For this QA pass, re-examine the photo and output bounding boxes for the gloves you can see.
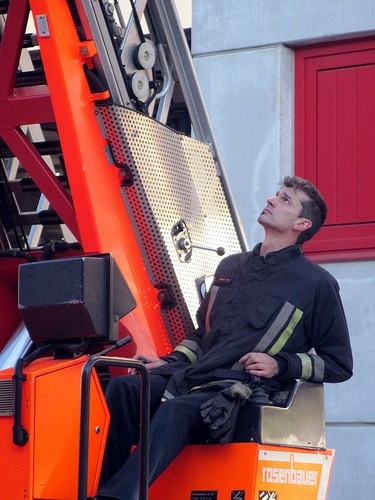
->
[199,383,252,445]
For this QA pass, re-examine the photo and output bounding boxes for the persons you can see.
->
[96,176,353,500]
